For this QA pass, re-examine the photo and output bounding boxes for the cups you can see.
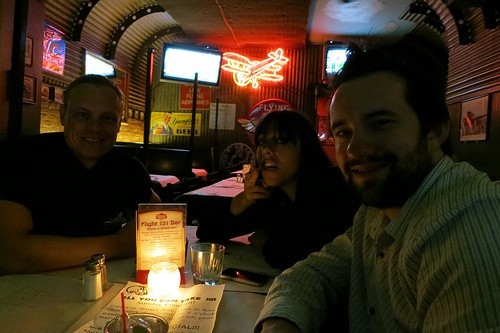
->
[104,313,169,333]
[148,262,181,296]
[242,164,251,182]
[189,244,225,285]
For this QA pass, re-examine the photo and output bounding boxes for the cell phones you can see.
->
[219,267,269,287]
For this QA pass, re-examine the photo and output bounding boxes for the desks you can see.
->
[181,175,248,223]
[0,225,282,333]
[149,173,181,204]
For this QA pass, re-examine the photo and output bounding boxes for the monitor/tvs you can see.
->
[321,41,350,84]
[83,51,114,80]
[159,44,222,88]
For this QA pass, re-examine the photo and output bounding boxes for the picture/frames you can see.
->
[25,35,34,67]
[22,74,38,105]
[459,93,490,142]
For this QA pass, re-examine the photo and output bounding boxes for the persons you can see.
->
[253,48,500,333]
[194,110,362,271]
[0,75,163,275]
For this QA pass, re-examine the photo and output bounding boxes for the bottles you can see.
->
[81,259,104,301]
[237,174,241,183]
[90,254,107,288]
